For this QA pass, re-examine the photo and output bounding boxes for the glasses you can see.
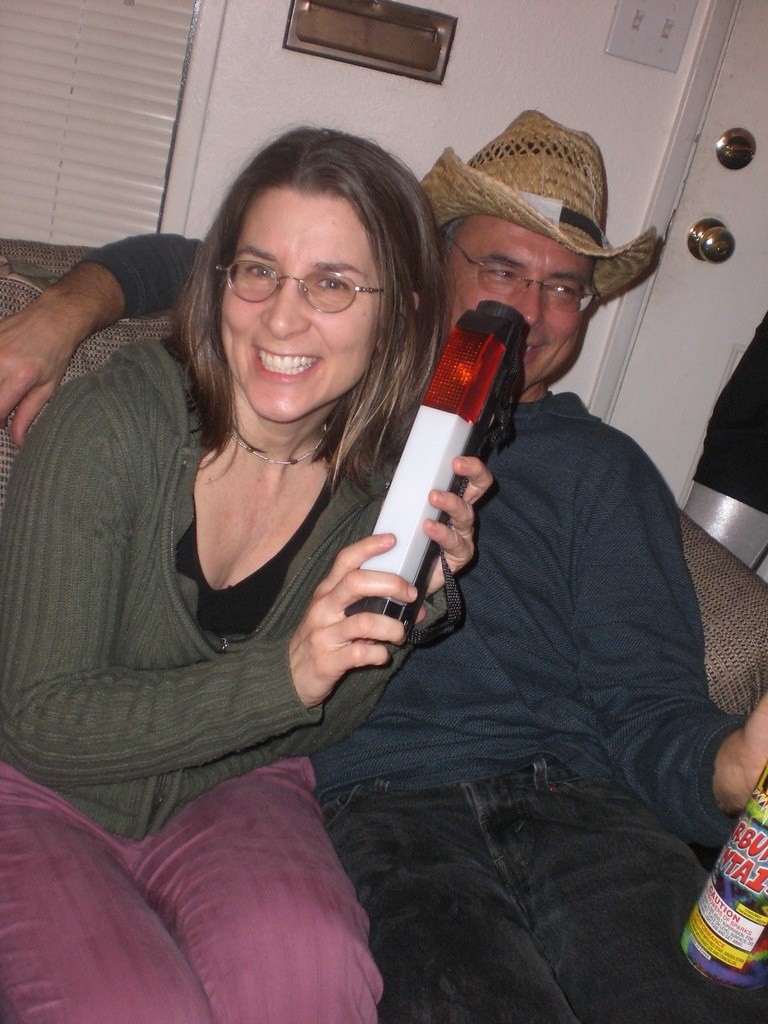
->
[446,234,601,312]
[215,260,383,314]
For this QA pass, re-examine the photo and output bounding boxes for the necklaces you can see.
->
[230,420,328,465]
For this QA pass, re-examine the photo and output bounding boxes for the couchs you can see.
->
[0,239,768,716]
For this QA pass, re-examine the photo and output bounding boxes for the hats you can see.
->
[420,110,657,300]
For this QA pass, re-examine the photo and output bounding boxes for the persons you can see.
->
[0,109,767,1024]
[0,126,494,1024]
